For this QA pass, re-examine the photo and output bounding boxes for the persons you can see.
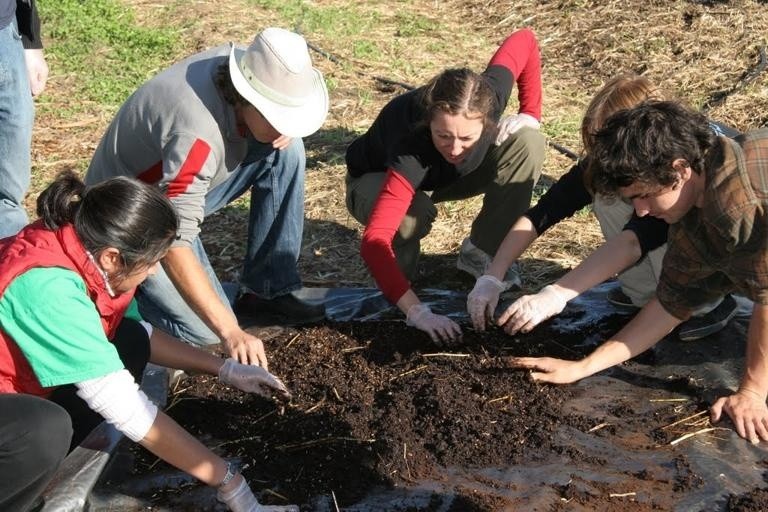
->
[347,24,547,346]
[0,1,50,241]
[463,72,741,345]
[511,88,768,447]
[2,167,300,511]
[83,27,330,370]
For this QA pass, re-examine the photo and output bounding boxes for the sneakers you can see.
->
[372,239,422,289]
[455,235,523,293]
[605,285,642,307]
[676,293,739,343]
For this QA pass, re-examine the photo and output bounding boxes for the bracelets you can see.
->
[213,459,238,491]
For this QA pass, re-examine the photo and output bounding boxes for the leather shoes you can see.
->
[231,288,328,326]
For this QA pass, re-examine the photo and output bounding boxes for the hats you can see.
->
[228,28,330,137]
